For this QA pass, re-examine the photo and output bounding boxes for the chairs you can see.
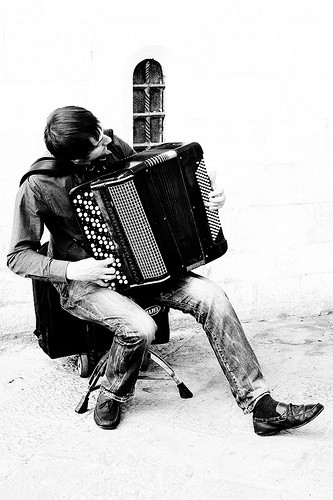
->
[33,242,194,414]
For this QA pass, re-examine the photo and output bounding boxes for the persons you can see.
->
[6,106,325,436]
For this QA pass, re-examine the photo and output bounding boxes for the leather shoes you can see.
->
[94,392,120,430]
[252,403,325,437]
